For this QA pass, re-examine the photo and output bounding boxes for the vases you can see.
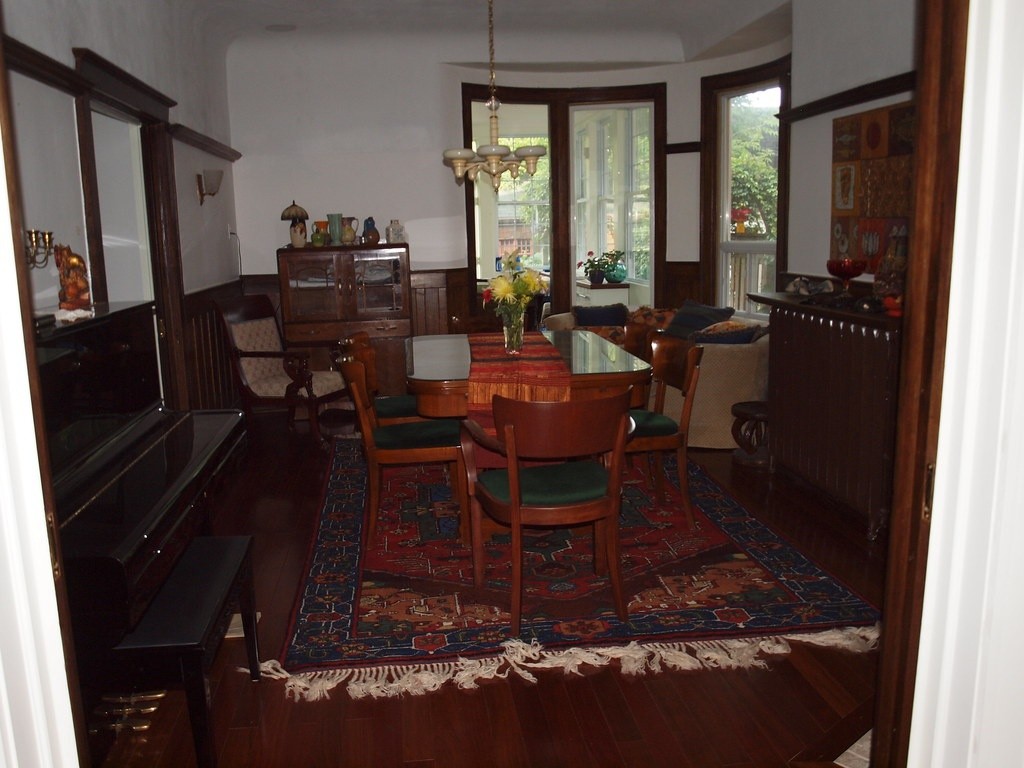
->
[498,303,526,355]
[589,270,605,284]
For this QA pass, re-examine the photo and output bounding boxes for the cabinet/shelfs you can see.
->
[576,280,630,306]
[274,243,411,396]
[748,291,902,541]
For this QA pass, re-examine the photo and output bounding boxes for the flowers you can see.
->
[578,251,606,276]
[483,249,548,348]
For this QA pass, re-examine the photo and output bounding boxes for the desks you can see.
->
[406,331,654,415]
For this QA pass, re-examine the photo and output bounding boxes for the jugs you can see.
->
[312,220,331,246]
[341,217,359,245]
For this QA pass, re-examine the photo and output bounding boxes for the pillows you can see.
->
[570,301,769,344]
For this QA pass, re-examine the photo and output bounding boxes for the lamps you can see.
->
[196,170,224,206]
[26,229,54,267]
[443,0,546,194]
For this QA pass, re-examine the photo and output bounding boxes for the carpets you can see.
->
[239,437,883,702]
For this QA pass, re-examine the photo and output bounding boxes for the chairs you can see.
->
[338,330,457,492]
[604,334,704,525]
[624,321,668,484]
[214,294,360,451]
[335,347,470,550]
[461,385,636,637]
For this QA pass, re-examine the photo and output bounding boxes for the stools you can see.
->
[731,401,771,461]
[114,533,262,768]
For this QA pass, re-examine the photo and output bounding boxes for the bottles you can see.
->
[326,213,344,247]
[386,220,406,244]
[364,217,380,245]
[311,228,323,248]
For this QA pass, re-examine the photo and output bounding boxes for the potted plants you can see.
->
[602,250,626,283]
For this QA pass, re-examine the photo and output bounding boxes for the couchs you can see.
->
[543,301,771,448]
